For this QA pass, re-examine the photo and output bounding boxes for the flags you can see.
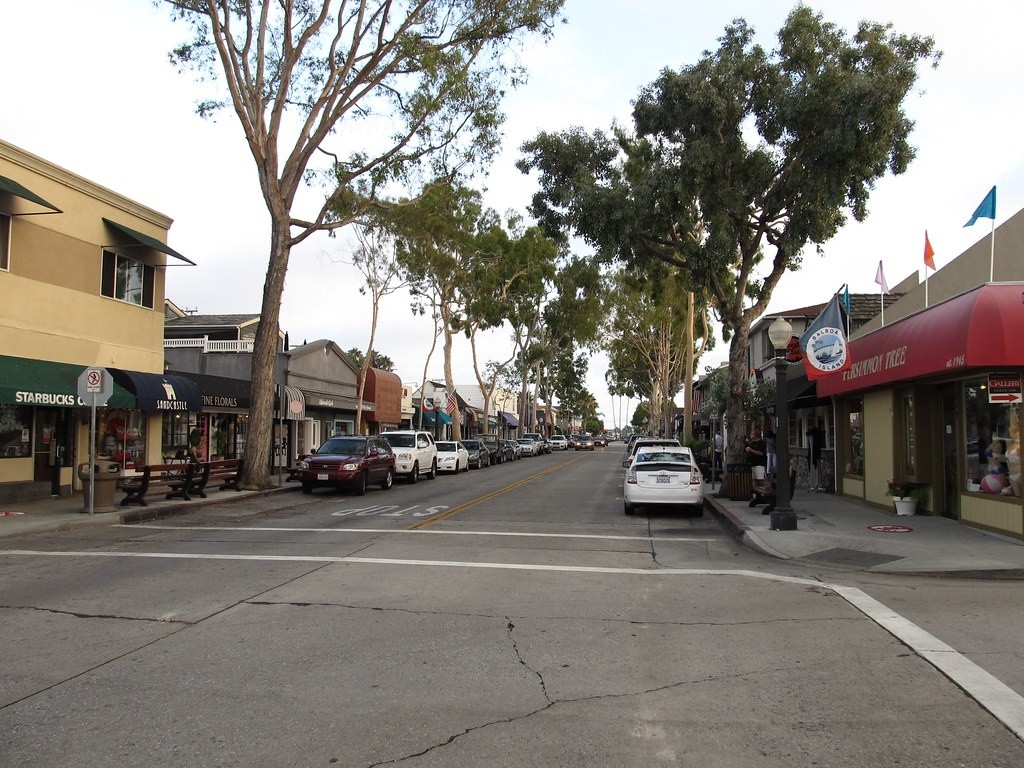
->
[962,186,996,227]
[799,296,853,383]
[875,261,890,296]
[841,285,851,316]
[924,230,937,272]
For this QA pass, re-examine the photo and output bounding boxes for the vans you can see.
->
[565,433,578,447]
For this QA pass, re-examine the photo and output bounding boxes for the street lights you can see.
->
[767,314,798,531]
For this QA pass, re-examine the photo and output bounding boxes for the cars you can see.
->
[461,440,490,470]
[508,439,522,460]
[500,439,514,462]
[543,438,553,454]
[576,435,595,451]
[622,445,706,519]
[434,441,469,475]
[516,438,538,457]
[626,435,653,453]
[550,435,568,450]
[626,438,680,472]
[593,436,608,447]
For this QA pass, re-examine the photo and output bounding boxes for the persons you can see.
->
[711,432,725,470]
[763,429,777,475]
[745,429,767,500]
[983,438,1012,495]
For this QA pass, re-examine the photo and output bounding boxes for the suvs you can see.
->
[474,434,500,464]
[299,433,395,496]
[524,433,544,455]
[380,429,437,483]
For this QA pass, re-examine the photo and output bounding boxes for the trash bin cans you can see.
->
[726,463,754,501]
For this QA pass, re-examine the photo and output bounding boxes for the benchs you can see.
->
[114,462,197,504]
[748,469,796,516]
[191,459,245,498]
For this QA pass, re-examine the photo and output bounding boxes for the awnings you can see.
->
[746,372,832,418]
[300,391,376,412]
[1,354,135,412]
[478,418,496,428]
[814,282,1024,399]
[498,411,520,428]
[436,409,453,425]
[102,367,203,416]
[270,383,305,421]
[411,405,442,428]
[165,370,281,410]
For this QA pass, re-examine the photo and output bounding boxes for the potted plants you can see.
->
[209,432,231,471]
[886,479,926,517]
[191,428,204,462]
